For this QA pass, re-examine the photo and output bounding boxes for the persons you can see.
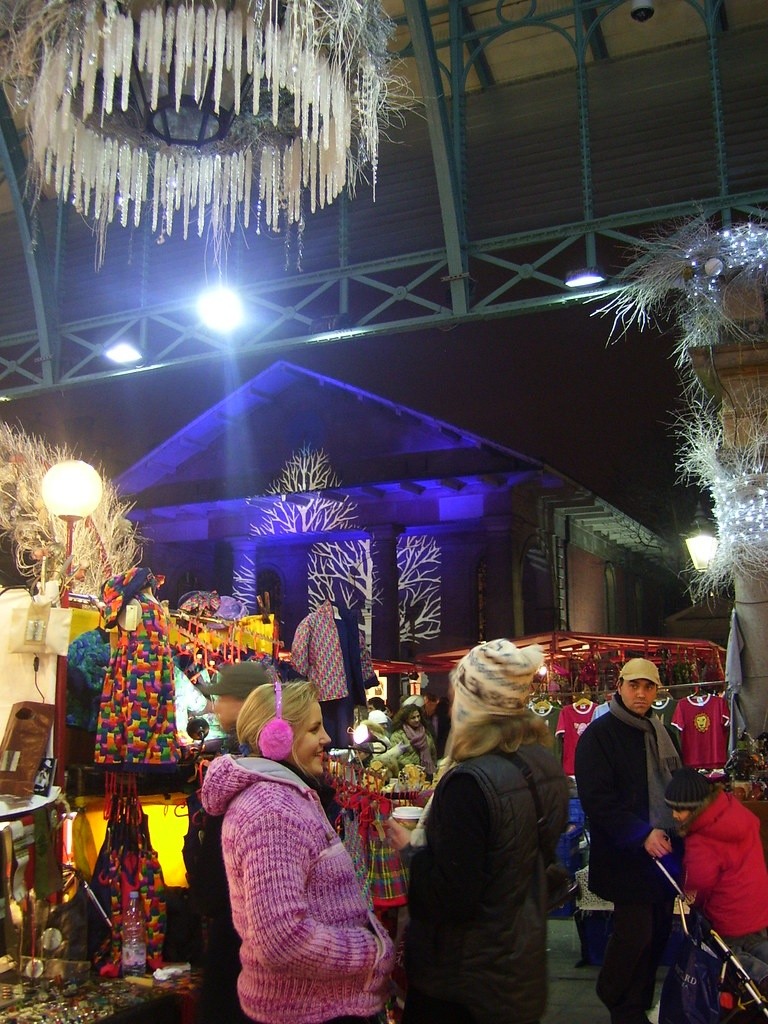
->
[395,637,570,1023]
[345,677,457,791]
[663,767,768,990]
[200,679,397,1024]
[572,657,686,1023]
[183,661,342,1024]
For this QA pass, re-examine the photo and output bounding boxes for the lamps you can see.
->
[0,0,446,276]
[41,459,104,589]
[681,501,720,573]
[102,335,146,369]
[564,265,606,288]
[305,313,354,335]
[187,716,210,763]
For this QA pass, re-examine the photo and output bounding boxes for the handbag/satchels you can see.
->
[0,700,56,796]
[658,933,724,1024]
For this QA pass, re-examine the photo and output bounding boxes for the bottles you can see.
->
[121,891,146,978]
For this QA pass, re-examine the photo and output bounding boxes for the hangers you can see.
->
[655,690,667,700]
[173,618,283,685]
[321,751,425,821]
[102,771,143,827]
[533,696,550,710]
[690,683,708,699]
[574,693,591,707]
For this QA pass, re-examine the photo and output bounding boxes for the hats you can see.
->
[616,657,661,688]
[400,695,425,708]
[201,661,268,697]
[450,639,544,712]
[664,768,710,810]
[368,710,388,723]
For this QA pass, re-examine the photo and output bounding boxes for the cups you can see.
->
[392,807,424,832]
